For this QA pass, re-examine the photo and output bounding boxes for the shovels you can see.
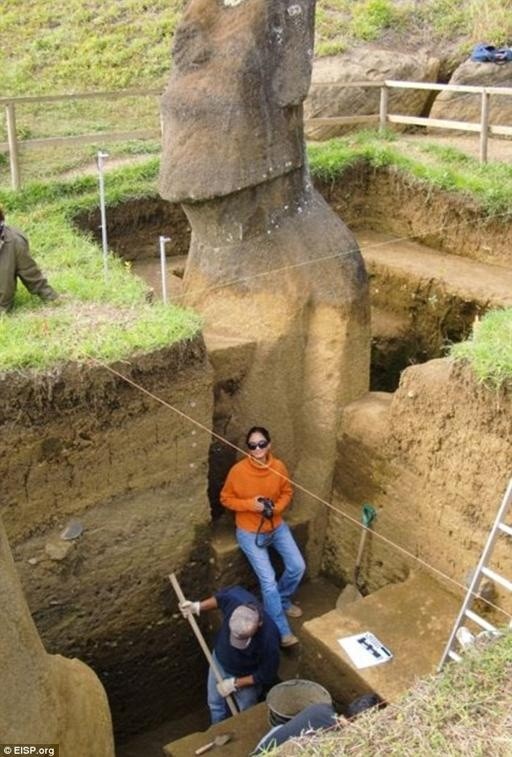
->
[336,505,375,610]
[195,733,231,755]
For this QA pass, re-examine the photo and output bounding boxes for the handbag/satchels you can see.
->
[471,45,512,65]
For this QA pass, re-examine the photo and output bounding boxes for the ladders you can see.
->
[437,477,512,672]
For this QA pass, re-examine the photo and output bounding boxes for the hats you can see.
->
[228,605,258,650]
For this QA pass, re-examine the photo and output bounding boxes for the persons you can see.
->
[219,425,308,649]
[0,206,60,315]
[249,694,385,756]
[179,581,283,724]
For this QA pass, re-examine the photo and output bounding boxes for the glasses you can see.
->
[248,441,268,449]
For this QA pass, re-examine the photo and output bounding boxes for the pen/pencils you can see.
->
[381,647,390,656]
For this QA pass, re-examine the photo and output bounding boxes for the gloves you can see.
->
[216,678,237,696]
[178,601,200,619]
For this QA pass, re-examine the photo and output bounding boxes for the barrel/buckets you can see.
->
[266,677,334,727]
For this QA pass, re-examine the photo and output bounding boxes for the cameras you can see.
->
[257,497,275,519]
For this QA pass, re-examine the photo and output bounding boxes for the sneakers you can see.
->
[285,605,302,617]
[280,635,299,647]
